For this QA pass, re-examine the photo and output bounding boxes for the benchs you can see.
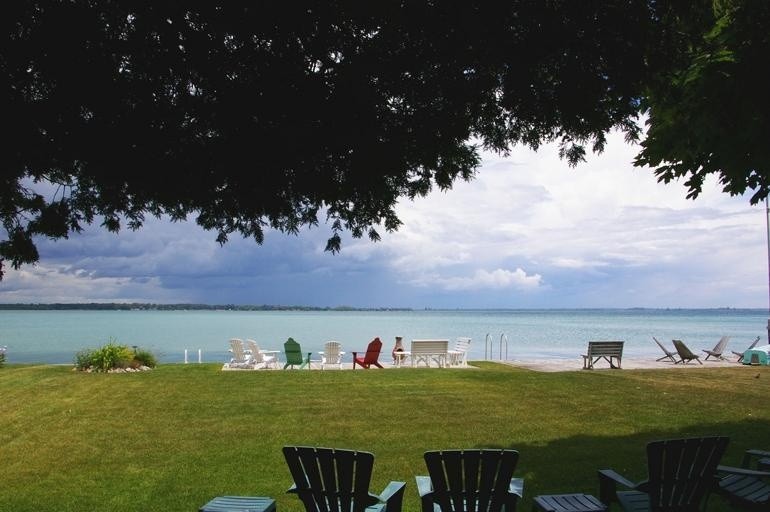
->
[410,340,449,367]
[580,340,625,370]
[447,337,472,367]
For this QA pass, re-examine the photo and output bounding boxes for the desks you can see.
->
[532,493,608,512]
[393,351,411,367]
[199,494,277,512]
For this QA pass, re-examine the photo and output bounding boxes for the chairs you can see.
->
[414,448,524,512]
[711,449,770,512]
[651,334,761,365]
[229,336,385,370]
[597,435,729,512]
[282,446,407,512]
